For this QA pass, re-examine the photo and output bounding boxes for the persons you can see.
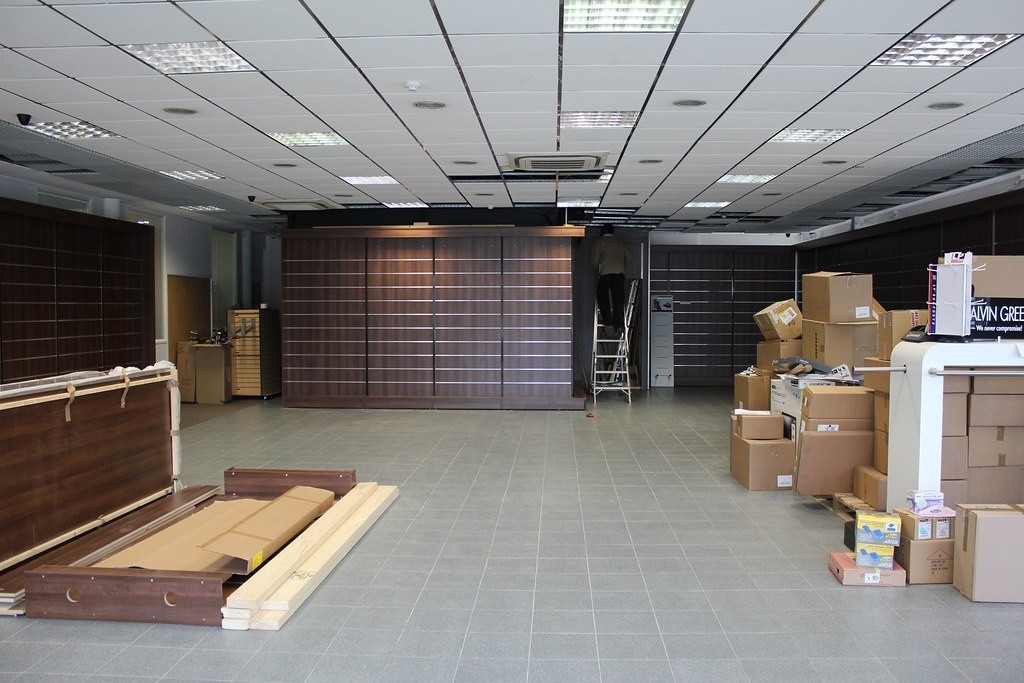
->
[591,223,632,339]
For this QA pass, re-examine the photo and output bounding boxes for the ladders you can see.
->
[609,279,639,390]
[590,278,631,404]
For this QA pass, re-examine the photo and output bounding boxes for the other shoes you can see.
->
[601,320,613,329]
[613,327,623,339]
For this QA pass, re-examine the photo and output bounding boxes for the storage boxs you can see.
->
[177,340,197,404]
[729,272,1024,604]
